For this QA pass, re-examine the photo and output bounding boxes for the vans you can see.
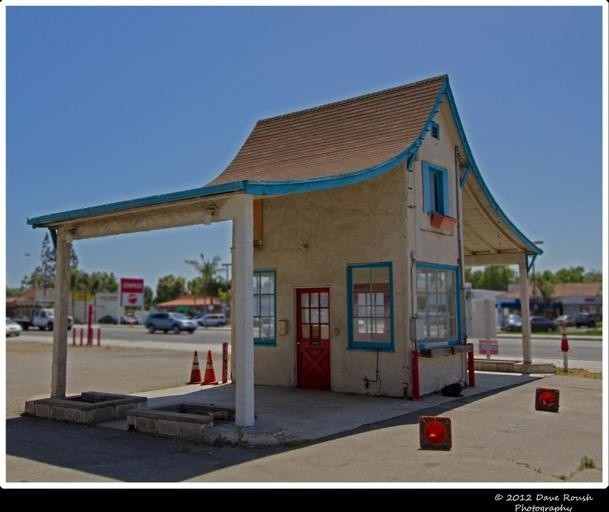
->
[196,313,226,326]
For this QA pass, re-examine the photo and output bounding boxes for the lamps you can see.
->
[203,204,219,226]
[64,226,77,244]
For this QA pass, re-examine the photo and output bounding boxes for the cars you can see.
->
[144,311,198,334]
[98,314,139,324]
[32,308,73,331]
[6,317,23,337]
[500,312,595,332]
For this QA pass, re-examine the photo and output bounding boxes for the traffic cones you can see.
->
[187,350,219,385]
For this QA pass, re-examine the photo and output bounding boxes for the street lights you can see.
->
[221,263,232,303]
